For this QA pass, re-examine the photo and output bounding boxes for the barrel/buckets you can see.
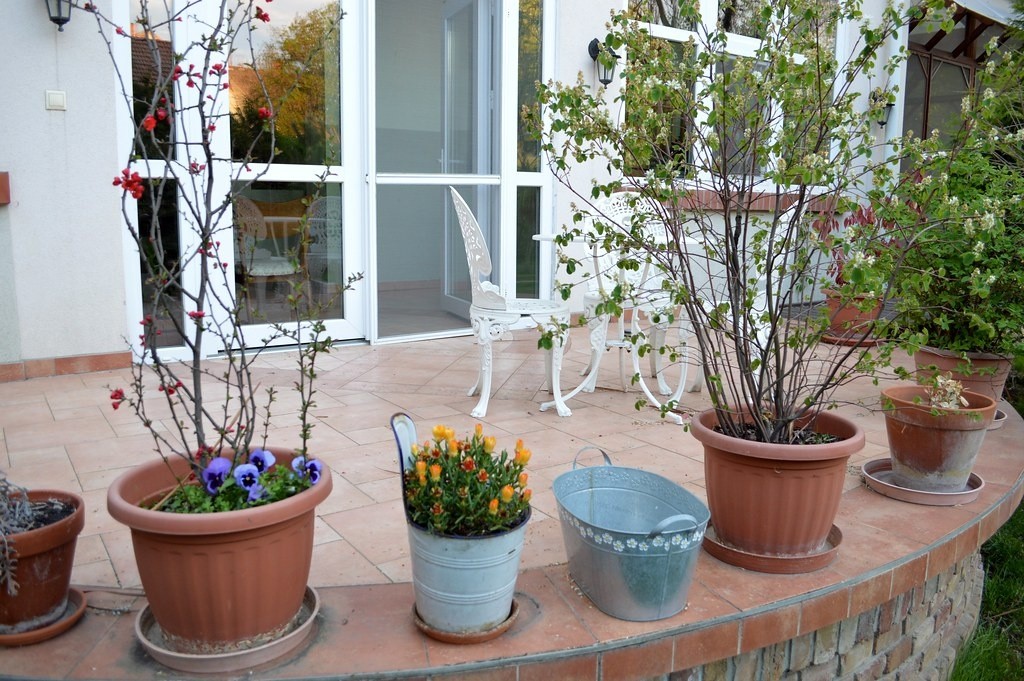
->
[553,447,710,622]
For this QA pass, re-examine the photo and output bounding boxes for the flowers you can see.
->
[63,0,367,513]
[402,423,532,536]
[520,0,1024,444]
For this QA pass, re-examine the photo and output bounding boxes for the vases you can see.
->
[406,492,532,646]
[106,445,334,681]
[688,403,867,575]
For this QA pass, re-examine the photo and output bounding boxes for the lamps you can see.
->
[589,37,622,90]
[45,0,72,32]
[868,91,896,129]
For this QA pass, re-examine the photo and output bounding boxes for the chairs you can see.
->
[449,186,572,419]
[582,190,678,396]
[236,196,319,325]
[664,195,810,410]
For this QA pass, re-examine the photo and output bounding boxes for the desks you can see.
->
[531,232,717,426]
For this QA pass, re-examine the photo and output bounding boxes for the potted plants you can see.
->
[812,170,927,348]
[861,371,996,507]
[886,67,1024,431]
[0,468,88,649]
